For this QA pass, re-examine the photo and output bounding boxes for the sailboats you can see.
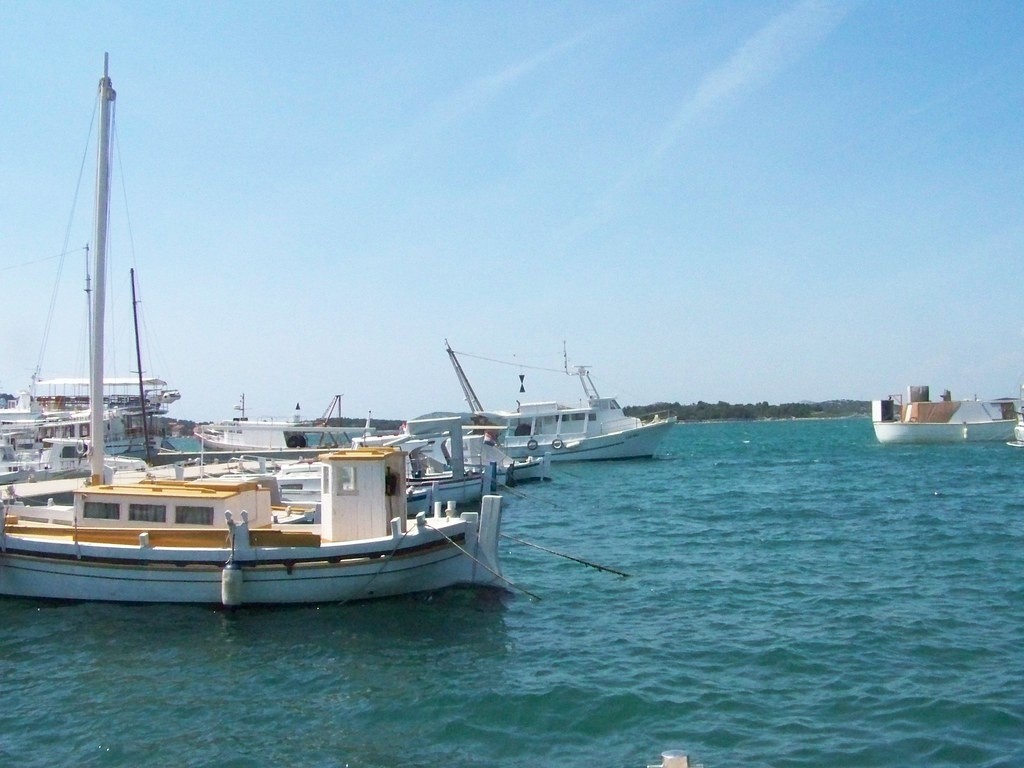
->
[0,49,521,612]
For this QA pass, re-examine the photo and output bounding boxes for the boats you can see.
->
[192,332,679,519]
[871,385,1017,443]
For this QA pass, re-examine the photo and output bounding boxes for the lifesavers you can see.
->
[552,438,563,450]
[75,440,85,455]
[527,439,538,450]
